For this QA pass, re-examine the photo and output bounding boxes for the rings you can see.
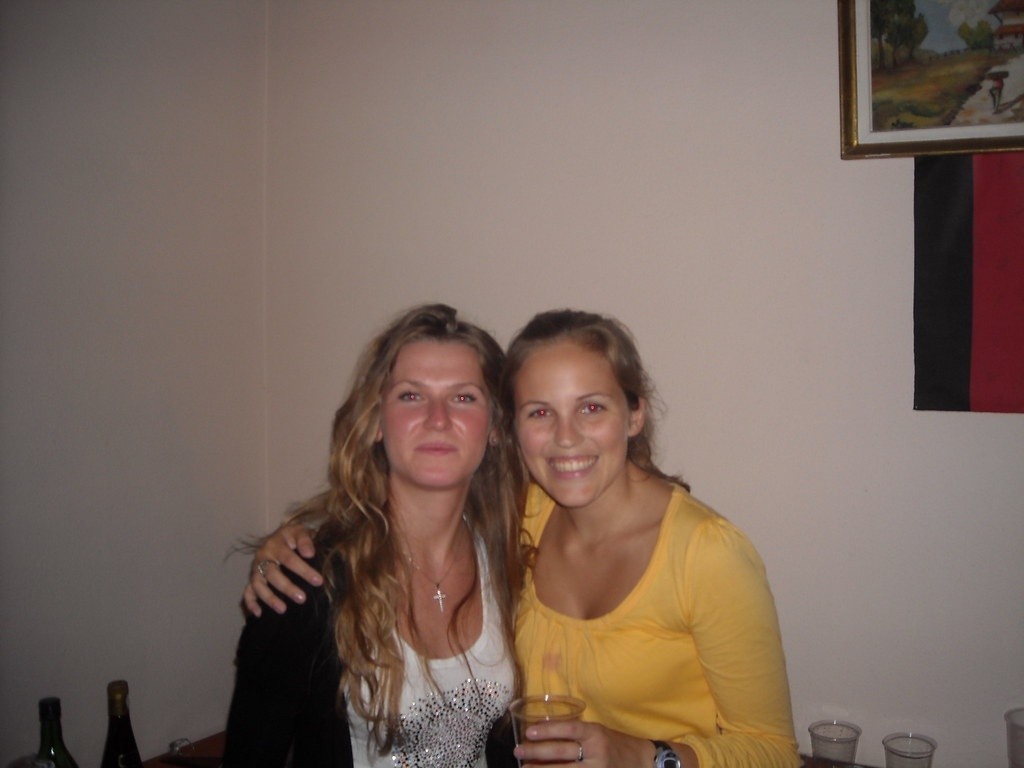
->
[575,741,584,762]
[256,559,280,577]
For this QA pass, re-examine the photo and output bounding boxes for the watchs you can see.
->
[649,739,682,768]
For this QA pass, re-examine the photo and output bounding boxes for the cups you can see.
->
[508,693,587,768]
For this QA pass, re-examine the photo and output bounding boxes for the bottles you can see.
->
[98,678,144,768]
[808,720,861,768]
[32,696,79,768]
[1004,707,1024,768]
[881,731,937,768]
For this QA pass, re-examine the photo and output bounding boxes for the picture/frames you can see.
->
[837,1,1024,161]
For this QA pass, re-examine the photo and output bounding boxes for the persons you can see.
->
[223,303,802,768]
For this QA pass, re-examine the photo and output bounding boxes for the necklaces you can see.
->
[402,520,466,613]
